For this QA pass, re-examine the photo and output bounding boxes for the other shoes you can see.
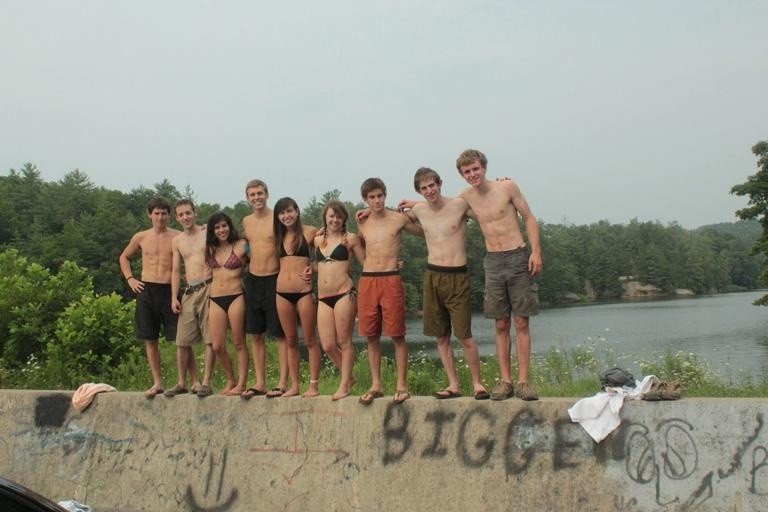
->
[146,385,165,397]
[164,384,188,396]
[197,385,213,396]
[191,384,202,394]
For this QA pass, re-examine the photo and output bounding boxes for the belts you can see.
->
[184,278,213,295]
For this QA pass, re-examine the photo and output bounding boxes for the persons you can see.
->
[239,181,315,399]
[312,201,406,400]
[274,195,319,397]
[204,212,252,396]
[118,196,210,397]
[396,149,543,401]
[354,166,512,399]
[163,200,216,397]
[314,177,425,404]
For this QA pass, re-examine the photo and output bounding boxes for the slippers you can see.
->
[239,387,265,400]
[392,390,411,402]
[359,390,383,403]
[474,390,490,400]
[435,390,462,399]
[266,388,285,398]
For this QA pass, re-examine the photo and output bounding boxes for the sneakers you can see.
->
[643,381,682,401]
[490,384,514,400]
[515,383,538,400]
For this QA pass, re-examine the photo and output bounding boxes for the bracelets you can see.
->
[126,273,134,282]
